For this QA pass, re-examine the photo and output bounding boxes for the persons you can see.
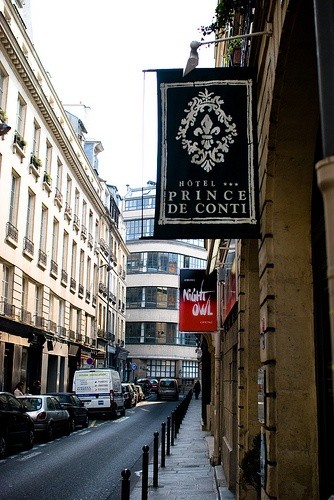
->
[13,382,24,396]
[193,381,201,400]
[27,381,42,395]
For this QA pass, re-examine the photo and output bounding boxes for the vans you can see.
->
[157,378,180,401]
[72,368,126,419]
[137,378,158,392]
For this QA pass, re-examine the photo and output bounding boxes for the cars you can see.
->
[15,395,71,442]
[0,391,35,459]
[43,393,89,431]
[121,382,146,408]
